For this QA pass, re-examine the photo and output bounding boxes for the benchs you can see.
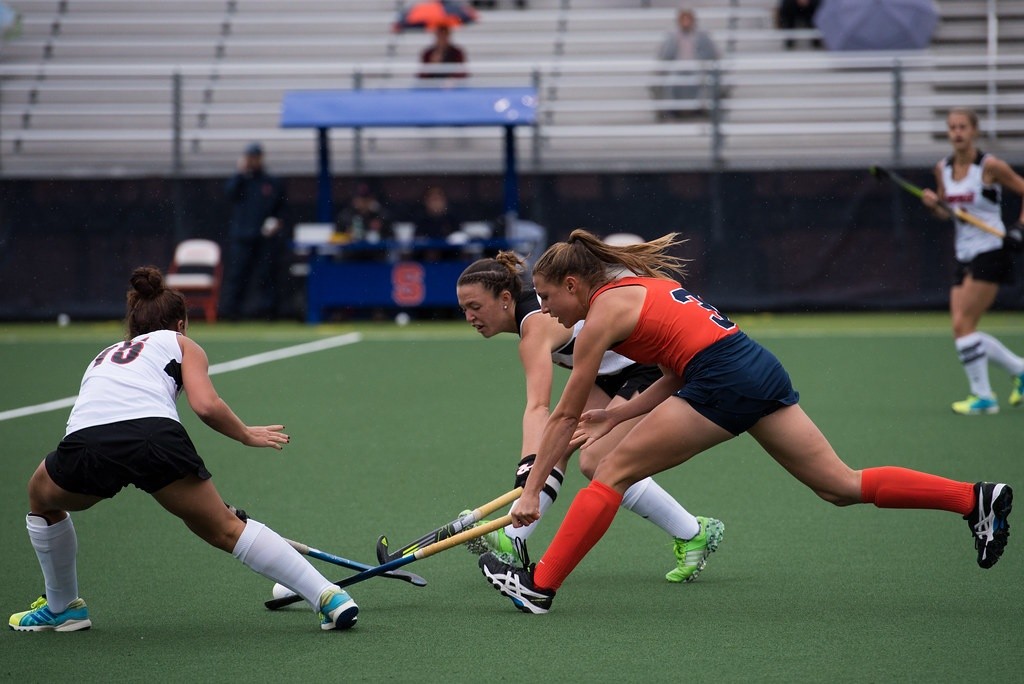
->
[290,223,547,297]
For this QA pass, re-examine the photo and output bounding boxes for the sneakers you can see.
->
[478,536,557,615]
[951,394,1000,415]
[962,481,1013,569]
[458,510,523,567]
[8,594,91,632]
[1009,370,1024,407]
[318,587,359,630]
[665,516,725,583]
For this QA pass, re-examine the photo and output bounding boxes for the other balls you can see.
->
[272,583,297,599]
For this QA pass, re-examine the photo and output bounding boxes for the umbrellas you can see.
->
[813,0,939,51]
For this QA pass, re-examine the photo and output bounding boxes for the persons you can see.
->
[456,252,724,583]
[419,24,466,78]
[479,229,1013,614]
[337,183,395,319]
[774,0,821,50]
[8,265,359,633]
[414,186,456,261]
[220,143,286,317]
[923,110,1024,415]
[648,12,728,118]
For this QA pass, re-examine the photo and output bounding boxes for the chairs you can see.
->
[163,237,223,323]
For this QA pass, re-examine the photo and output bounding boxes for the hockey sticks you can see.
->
[377,484,524,571]
[282,537,428,587]
[264,514,513,610]
[870,160,1006,239]
